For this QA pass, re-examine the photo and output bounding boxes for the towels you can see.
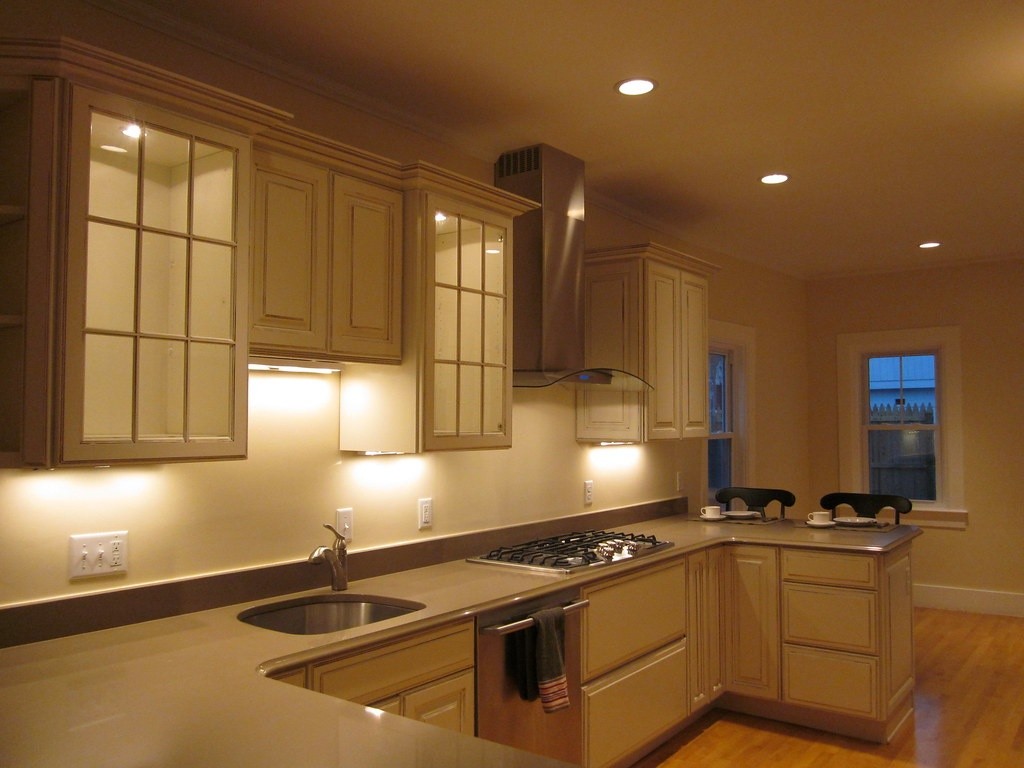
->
[516,604,571,713]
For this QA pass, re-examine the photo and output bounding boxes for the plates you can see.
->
[806,521,835,527]
[721,512,761,518]
[834,516,877,526]
[700,515,726,521]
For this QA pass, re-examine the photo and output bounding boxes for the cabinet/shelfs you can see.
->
[781,542,916,720]
[719,541,778,704]
[580,557,691,768]
[1,35,296,476]
[687,546,724,709]
[251,123,407,378]
[337,158,542,464]
[578,241,722,448]
[307,615,476,740]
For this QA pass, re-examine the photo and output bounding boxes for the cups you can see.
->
[808,511,829,523]
[701,506,720,516]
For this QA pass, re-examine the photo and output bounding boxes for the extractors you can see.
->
[495,144,653,392]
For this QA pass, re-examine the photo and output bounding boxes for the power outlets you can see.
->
[70,530,129,584]
[418,498,434,531]
[583,480,593,506]
[677,471,683,491]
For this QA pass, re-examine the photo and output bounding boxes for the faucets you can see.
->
[308,523,352,591]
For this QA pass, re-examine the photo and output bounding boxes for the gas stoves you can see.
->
[464,524,674,576]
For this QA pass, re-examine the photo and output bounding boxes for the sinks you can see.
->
[236,591,427,636]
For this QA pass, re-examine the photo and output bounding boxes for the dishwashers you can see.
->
[476,587,589,768]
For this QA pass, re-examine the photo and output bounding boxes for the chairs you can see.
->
[715,486,796,519]
[821,490,912,525]
